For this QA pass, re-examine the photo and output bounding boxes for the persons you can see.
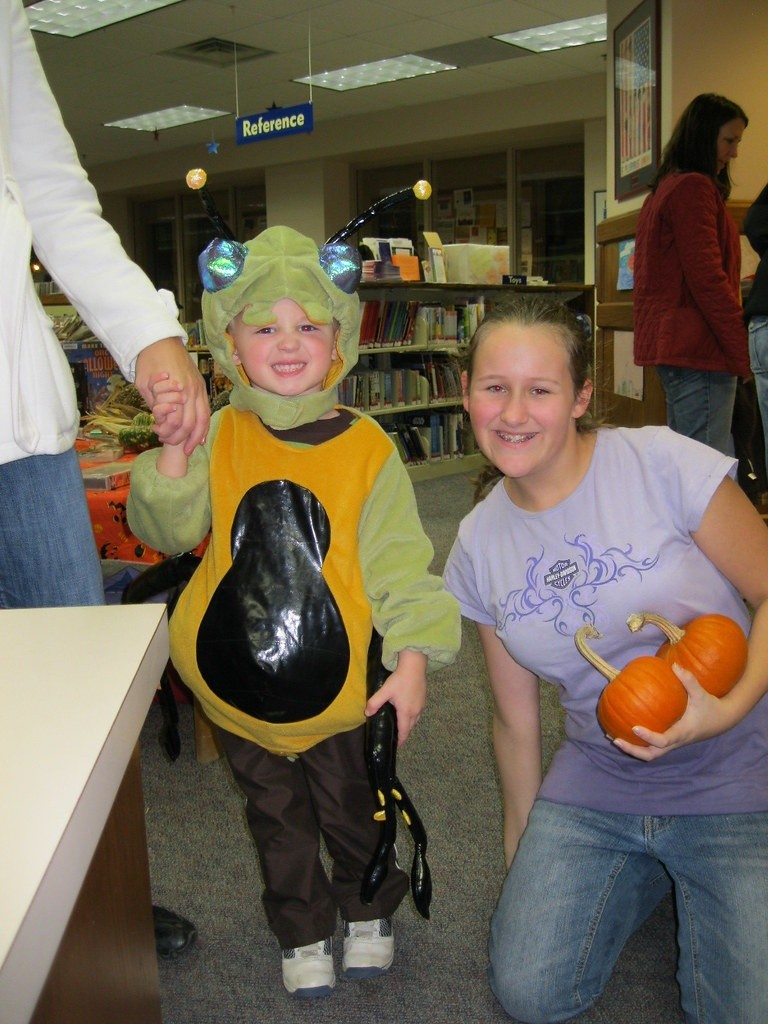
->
[126,225,462,998]
[633,92,768,482]
[0,0,211,956]
[443,296,768,1024]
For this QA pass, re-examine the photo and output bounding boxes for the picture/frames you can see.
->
[612,0,662,199]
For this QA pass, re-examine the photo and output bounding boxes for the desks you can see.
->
[0,591,171,1024]
[72,439,210,563]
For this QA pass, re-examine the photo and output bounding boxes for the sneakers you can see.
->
[282,935,334,995]
[341,919,395,977]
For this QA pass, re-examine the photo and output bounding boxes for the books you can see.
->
[49,312,100,341]
[34,281,64,297]
[182,318,234,405]
[338,238,549,469]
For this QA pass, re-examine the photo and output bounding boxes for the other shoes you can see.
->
[153,905,195,958]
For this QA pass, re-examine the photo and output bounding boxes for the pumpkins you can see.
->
[574,626,687,748]
[627,612,747,698]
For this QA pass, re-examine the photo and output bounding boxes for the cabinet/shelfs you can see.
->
[60,280,599,488]
